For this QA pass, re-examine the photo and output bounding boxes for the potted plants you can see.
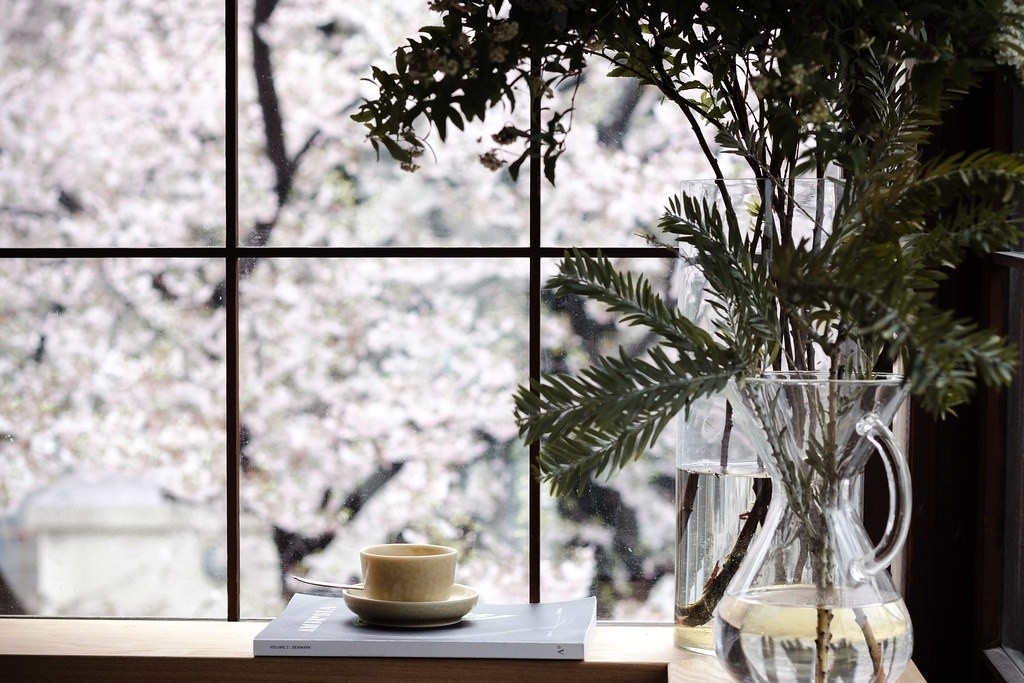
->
[350,0,1024,683]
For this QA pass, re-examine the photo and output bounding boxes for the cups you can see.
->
[359,544,459,602]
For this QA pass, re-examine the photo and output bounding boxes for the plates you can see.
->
[343,581,481,627]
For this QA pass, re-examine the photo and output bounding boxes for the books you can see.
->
[254,592,597,659]
